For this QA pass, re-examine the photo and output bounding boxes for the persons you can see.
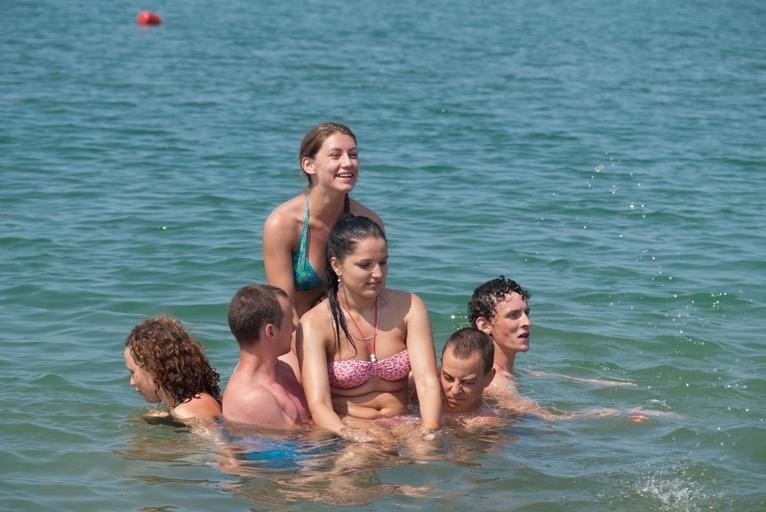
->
[222,284,342,473]
[294,212,445,503]
[123,312,252,474]
[261,120,386,382]
[439,327,505,436]
[467,274,688,422]
